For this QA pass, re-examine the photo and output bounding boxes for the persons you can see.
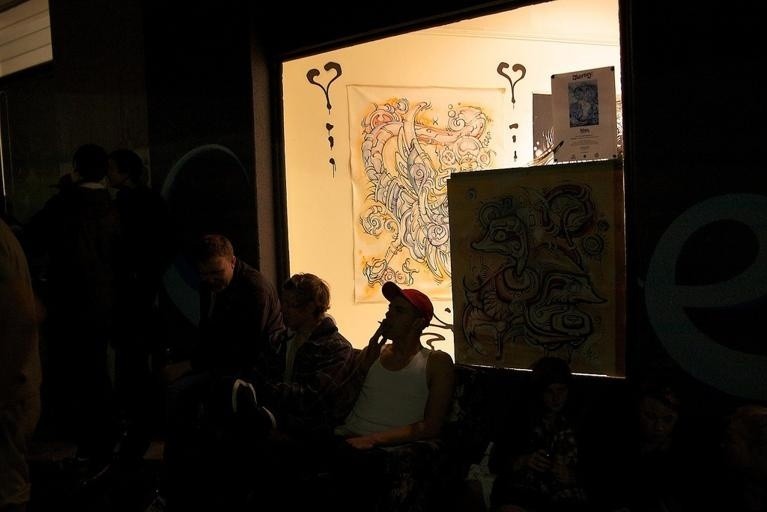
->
[39,143,112,225]
[193,234,285,348]
[727,406,767,481]
[103,147,149,198]
[323,281,454,459]
[0,217,46,512]
[490,356,607,512]
[632,378,683,443]
[217,273,355,436]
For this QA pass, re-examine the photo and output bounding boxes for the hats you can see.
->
[382,282,433,326]
